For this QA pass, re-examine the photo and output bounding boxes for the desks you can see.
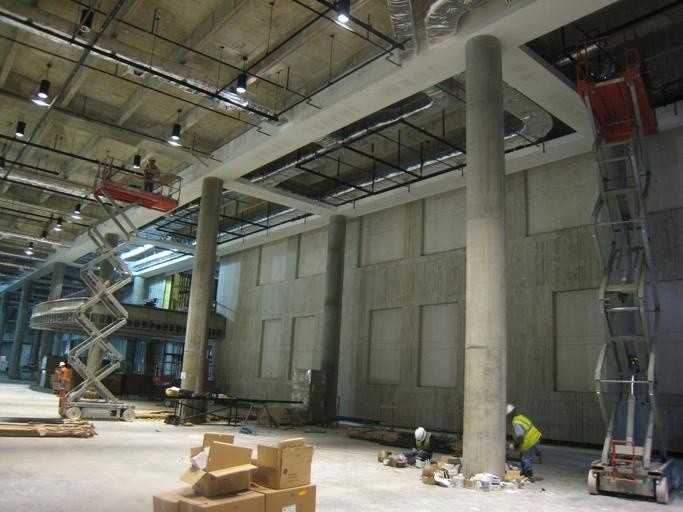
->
[162,395,236,427]
[237,398,303,429]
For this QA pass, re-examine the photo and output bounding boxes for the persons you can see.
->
[399,427,436,465]
[54,362,67,397]
[143,156,160,193]
[506,403,542,483]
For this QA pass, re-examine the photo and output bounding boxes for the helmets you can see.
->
[415,426,426,442]
[507,404,515,416]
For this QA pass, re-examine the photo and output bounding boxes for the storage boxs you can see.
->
[151,431,317,512]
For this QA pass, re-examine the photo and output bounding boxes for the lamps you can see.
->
[57,217,63,227]
[74,203,81,214]
[133,155,141,170]
[336,2,351,23]
[171,123,181,141]
[236,72,247,94]
[15,121,26,137]
[40,230,47,242]
[38,79,51,99]
[78,7,95,33]
[29,241,33,250]
